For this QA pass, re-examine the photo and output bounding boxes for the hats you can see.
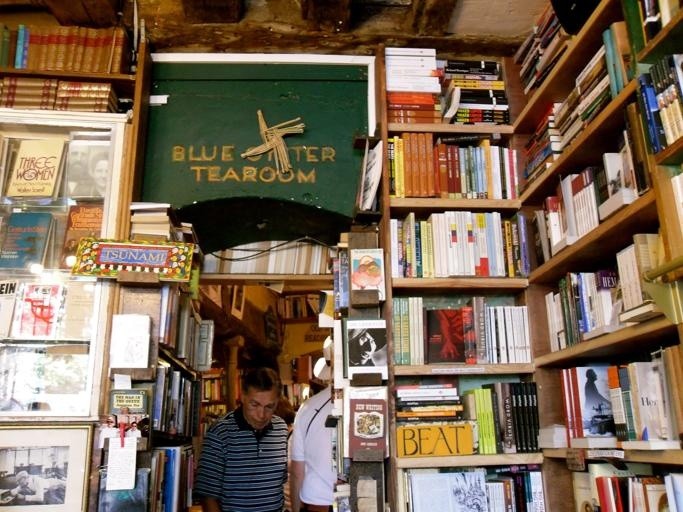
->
[313,336,333,380]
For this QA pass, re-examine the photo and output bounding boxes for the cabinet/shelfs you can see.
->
[197,363,245,434]
[99,192,206,512]
[371,46,548,512]
[0,27,146,512]
[509,0,682,512]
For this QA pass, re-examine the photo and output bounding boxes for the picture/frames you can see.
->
[0,425,91,512]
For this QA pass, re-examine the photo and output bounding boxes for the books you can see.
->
[514,0,682,512]
[2,19,131,203]
[1,201,214,511]
[331,47,546,511]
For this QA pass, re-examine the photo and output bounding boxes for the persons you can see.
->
[192,365,289,512]
[286,329,335,512]
